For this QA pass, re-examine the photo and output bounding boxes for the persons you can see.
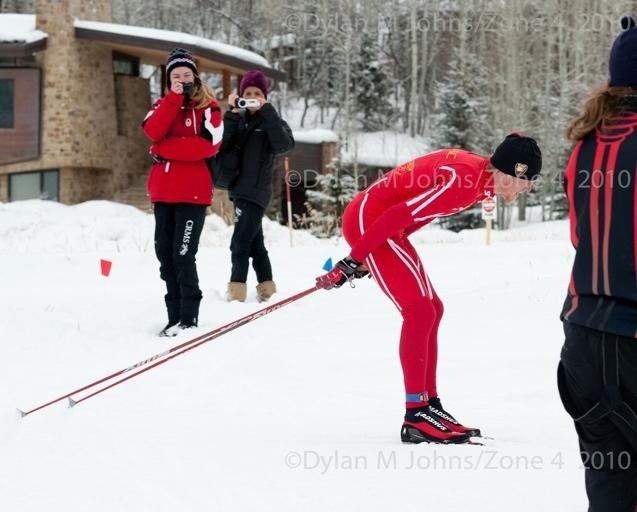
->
[139,45,224,338]
[314,132,543,446]
[221,70,297,305]
[555,15,637,511]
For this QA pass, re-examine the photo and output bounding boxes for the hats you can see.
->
[240,70,267,97]
[165,46,197,75]
[490,132,542,180]
[608,27,637,89]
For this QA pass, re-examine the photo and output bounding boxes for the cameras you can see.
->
[235,97,260,109]
[181,81,194,98]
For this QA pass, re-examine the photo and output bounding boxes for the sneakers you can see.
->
[400,398,480,445]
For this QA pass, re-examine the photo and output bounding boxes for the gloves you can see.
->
[316,257,369,290]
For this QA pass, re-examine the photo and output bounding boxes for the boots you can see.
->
[161,292,202,336]
[256,280,276,303]
[226,282,247,302]
